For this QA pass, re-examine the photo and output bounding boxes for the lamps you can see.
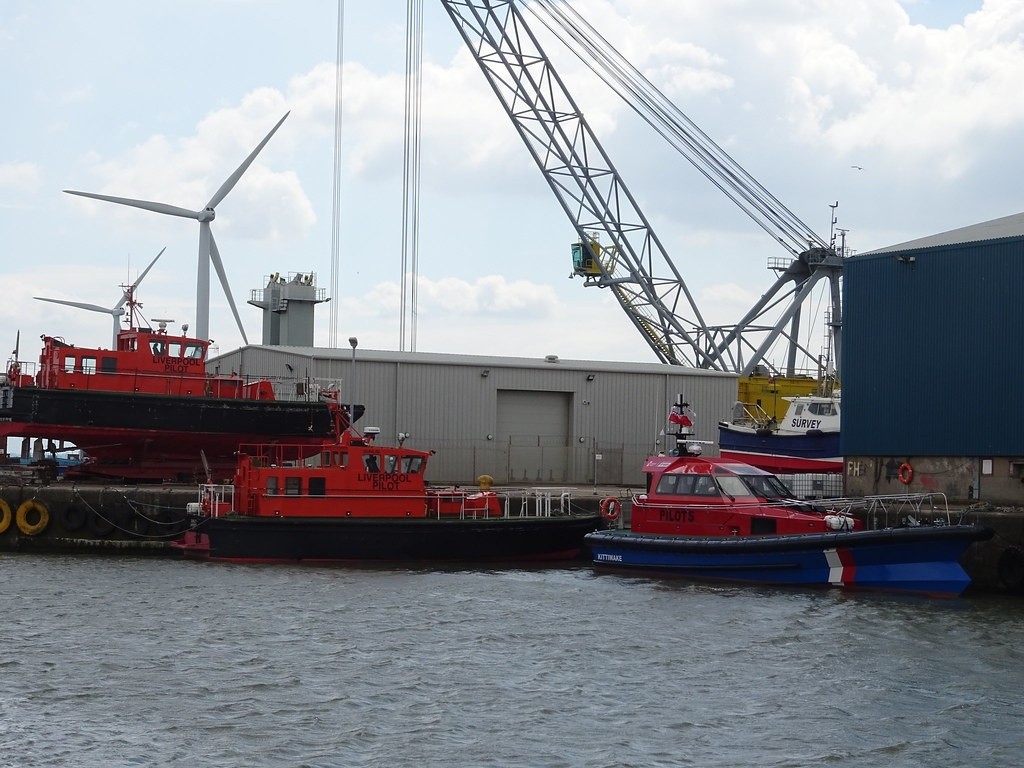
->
[481,369,489,377]
[891,253,910,262]
[586,375,594,381]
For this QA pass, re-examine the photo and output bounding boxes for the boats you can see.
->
[718,352,845,478]
[582,393,996,598]
[164,424,622,565]
[0,249,366,484]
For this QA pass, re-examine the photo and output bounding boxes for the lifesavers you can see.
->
[58,502,87,532]
[88,506,116,538]
[898,463,913,484]
[0,499,11,533]
[478,475,493,491]
[15,498,51,538]
[602,498,621,519]
[120,513,150,539]
[155,510,182,540]
